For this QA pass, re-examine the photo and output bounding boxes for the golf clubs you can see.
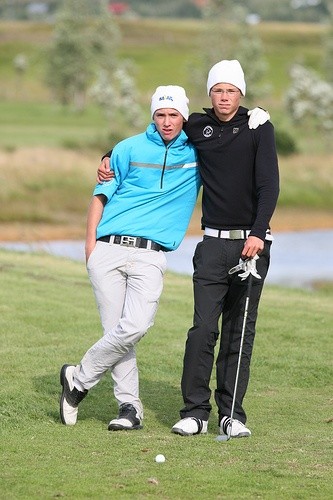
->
[216,260,257,442]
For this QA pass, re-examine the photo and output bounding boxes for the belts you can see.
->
[204,225,274,244]
[97,233,171,253]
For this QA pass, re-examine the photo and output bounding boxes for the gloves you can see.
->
[246,105,270,131]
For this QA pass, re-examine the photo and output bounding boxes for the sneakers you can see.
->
[56,364,89,428]
[106,401,145,433]
[171,414,210,437]
[217,412,251,440]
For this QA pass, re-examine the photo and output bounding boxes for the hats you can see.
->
[150,84,190,121]
[206,58,246,98]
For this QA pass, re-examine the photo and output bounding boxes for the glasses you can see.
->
[212,87,238,96]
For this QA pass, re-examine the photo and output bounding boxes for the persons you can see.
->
[60,85,270,431]
[96,59,280,438]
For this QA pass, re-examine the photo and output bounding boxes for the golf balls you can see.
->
[155,454,165,463]
